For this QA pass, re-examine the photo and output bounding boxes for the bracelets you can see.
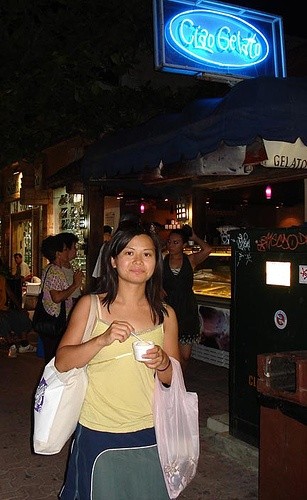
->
[157,359,171,371]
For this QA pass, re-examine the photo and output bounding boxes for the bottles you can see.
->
[74,268,89,291]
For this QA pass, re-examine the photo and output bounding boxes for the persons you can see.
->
[13,253,31,278]
[53,224,180,500]
[39,233,86,363]
[162,225,212,360]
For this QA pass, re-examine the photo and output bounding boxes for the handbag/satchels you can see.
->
[32,300,67,340]
[32,293,98,455]
[154,357,199,498]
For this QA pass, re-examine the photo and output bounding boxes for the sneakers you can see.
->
[8,345,17,358]
[18,344,37,353]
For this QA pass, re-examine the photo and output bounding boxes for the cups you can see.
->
[164,220,181,230]
[132,340,155,363]
